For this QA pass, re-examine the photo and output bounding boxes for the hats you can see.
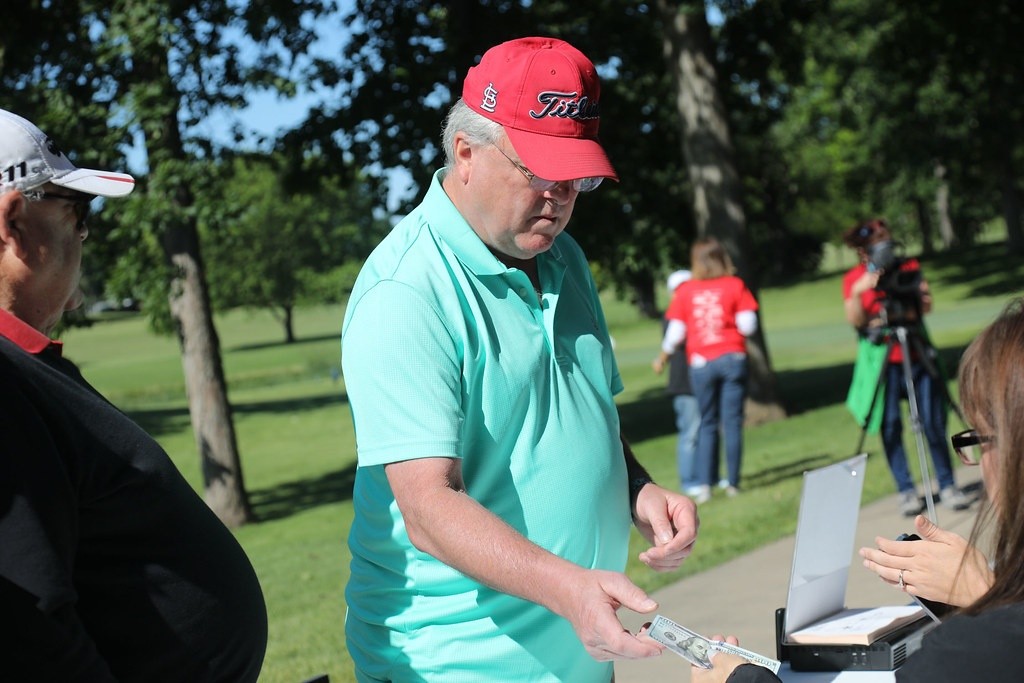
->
[462,37,619,183]
[0,109,135,198]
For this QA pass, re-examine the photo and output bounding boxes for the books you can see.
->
[781,454,928,647]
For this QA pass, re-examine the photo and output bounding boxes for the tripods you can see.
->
[855,295,969,526]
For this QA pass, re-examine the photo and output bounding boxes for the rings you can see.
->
[898,568,907,589]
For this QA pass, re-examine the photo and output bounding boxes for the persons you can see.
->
[342,35,702,681]
[662,266,700,498]
[1,107,271,683]
[647,231,760,502]
[690,298,1024,683]
[843,216,972,519]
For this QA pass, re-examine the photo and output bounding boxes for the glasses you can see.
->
[23,190,91,231]
[488,138,605,193]
[951,429,997,465]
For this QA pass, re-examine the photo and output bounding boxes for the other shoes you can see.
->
[939,484,969,509]
[902,491,924,515]
